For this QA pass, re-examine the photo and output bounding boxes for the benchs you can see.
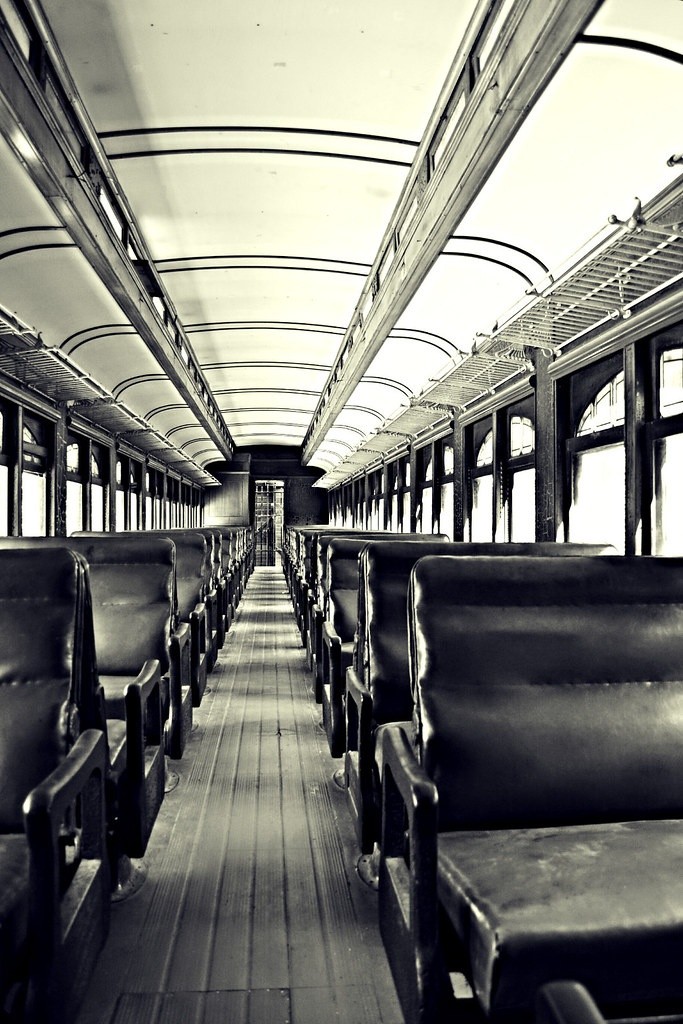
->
[0,523,255,1024]
[279,520,682,1023]
